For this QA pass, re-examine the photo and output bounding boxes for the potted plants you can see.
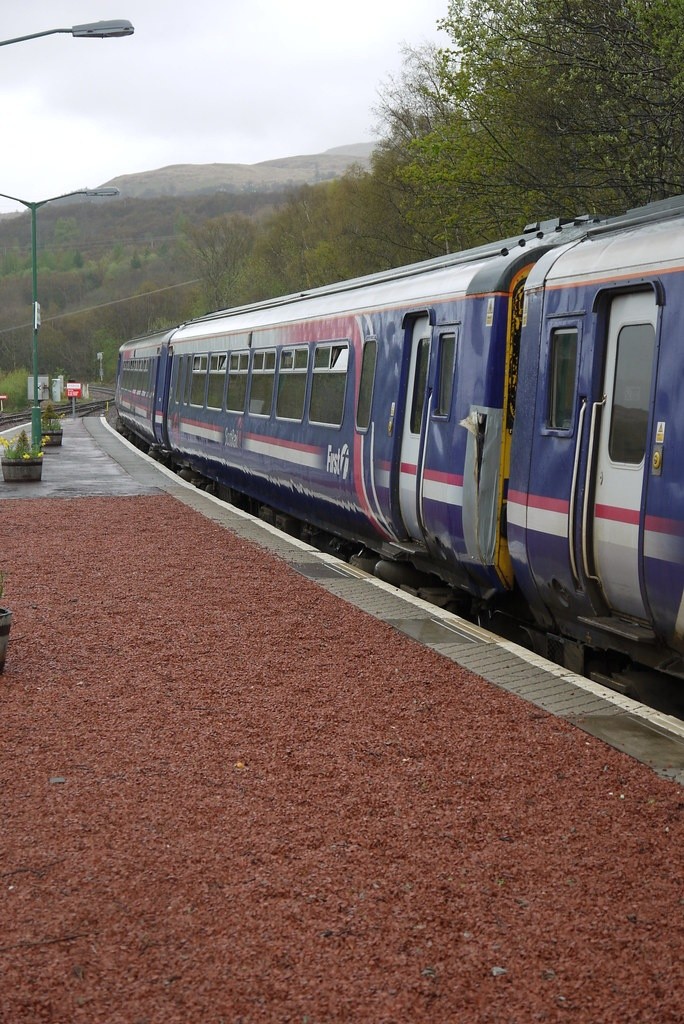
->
[0,567,13,678]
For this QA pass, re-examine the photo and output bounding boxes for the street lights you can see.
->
[0,186,119,473]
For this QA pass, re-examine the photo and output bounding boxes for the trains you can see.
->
[115,192,684,708]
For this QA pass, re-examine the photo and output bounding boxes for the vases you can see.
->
[1,457,45,482]
[41,429,63,446]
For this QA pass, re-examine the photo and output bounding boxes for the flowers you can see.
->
[0,430,50,462]
[41,403,65,430]
